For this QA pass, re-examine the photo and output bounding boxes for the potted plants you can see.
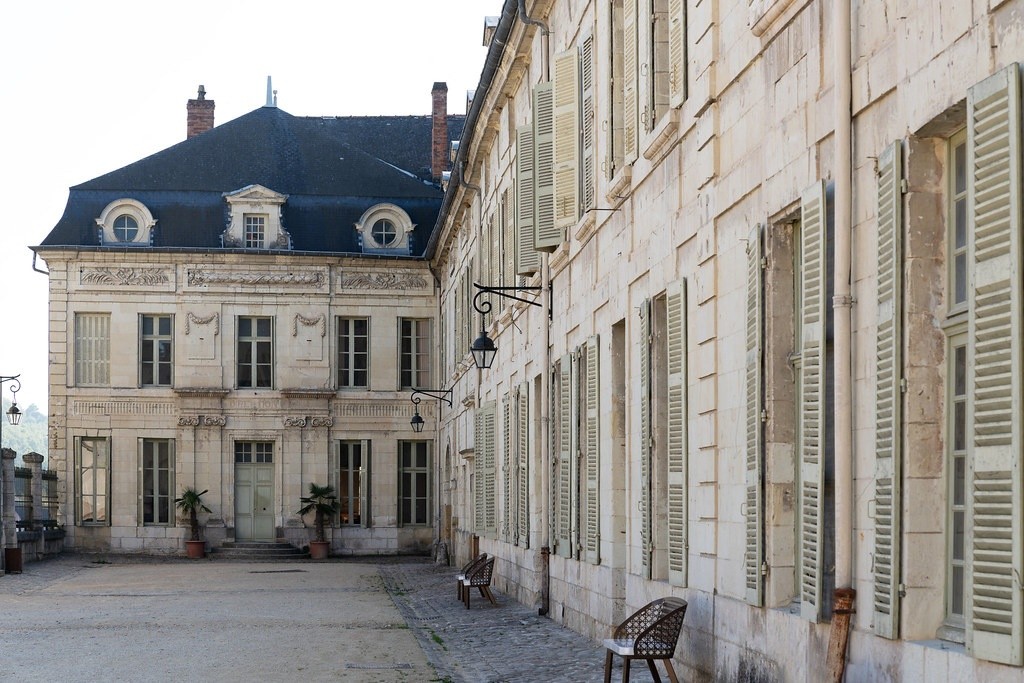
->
[172,485,214,559]
[296,481,342,560]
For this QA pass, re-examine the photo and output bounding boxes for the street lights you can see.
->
[0,374,22,449]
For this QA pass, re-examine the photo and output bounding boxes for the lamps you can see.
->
[409,387,453,432]
[469,278,554,370]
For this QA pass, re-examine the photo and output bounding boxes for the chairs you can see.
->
[600,596,688,683]
[462,556,498,610]
[454,552,488,602]
